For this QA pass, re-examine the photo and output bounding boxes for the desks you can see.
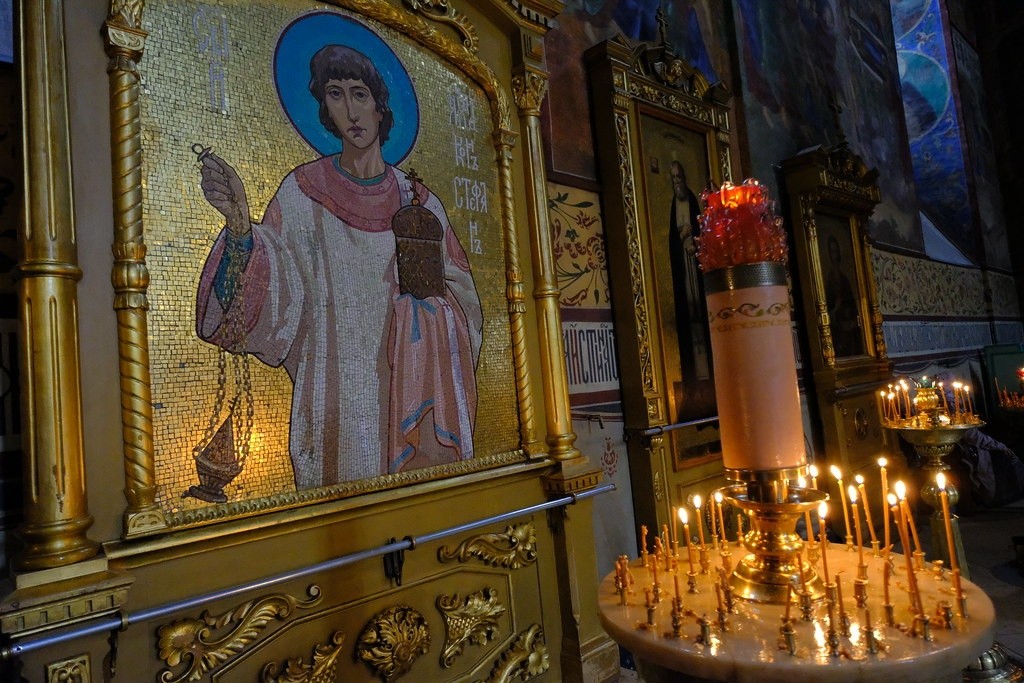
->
[595,538,998,683]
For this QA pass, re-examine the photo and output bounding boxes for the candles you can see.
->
[879,375,973,423]
[614,455,965,634]
